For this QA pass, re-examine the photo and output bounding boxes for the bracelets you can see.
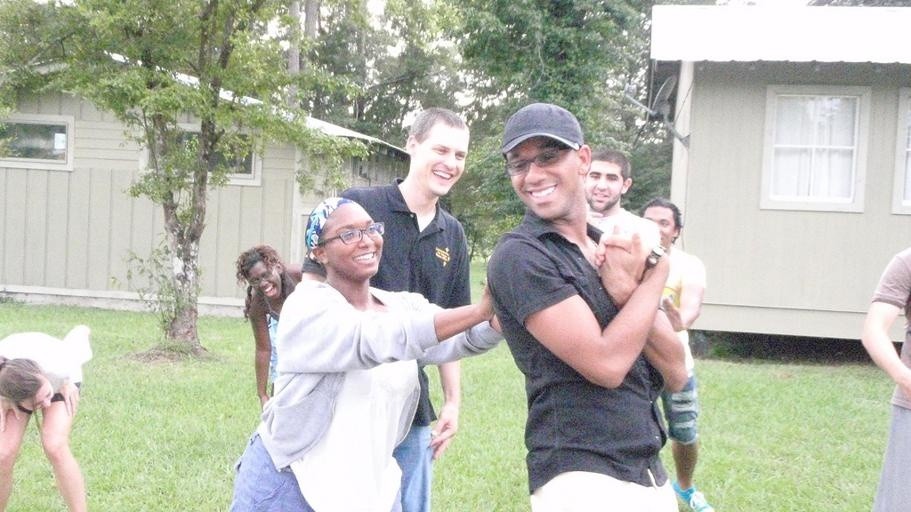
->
[640,247,663,282]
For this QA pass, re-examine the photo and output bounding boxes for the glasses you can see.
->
[504,146,569,176]
[317,221,385,246]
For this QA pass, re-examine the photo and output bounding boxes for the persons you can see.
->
[332,107,471,511]
[585,147,662,259]
[232,195,506,512]
[637,196,715,512]
[0,322,93,511]
[859,246,911,511]
[487,101,692,512]
[234,244,302,412]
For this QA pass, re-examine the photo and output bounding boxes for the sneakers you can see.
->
[672,480,717,511]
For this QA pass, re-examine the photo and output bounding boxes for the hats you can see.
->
[502,102,584,156]
